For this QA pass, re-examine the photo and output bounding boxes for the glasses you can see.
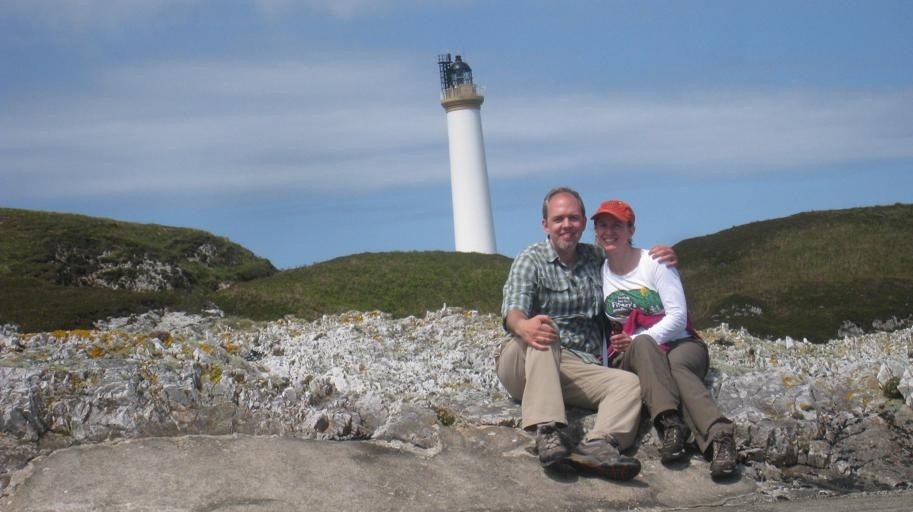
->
[610,321,622,335]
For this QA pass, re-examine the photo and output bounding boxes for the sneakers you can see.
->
[658,418,695,466]
[564,433,642,482]
[533,420,568,468]
[710,431,739,479]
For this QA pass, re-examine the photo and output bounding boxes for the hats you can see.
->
[590,199,636,225]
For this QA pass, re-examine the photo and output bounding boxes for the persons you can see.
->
[495,187,678,482]
[590,200,739,482]
